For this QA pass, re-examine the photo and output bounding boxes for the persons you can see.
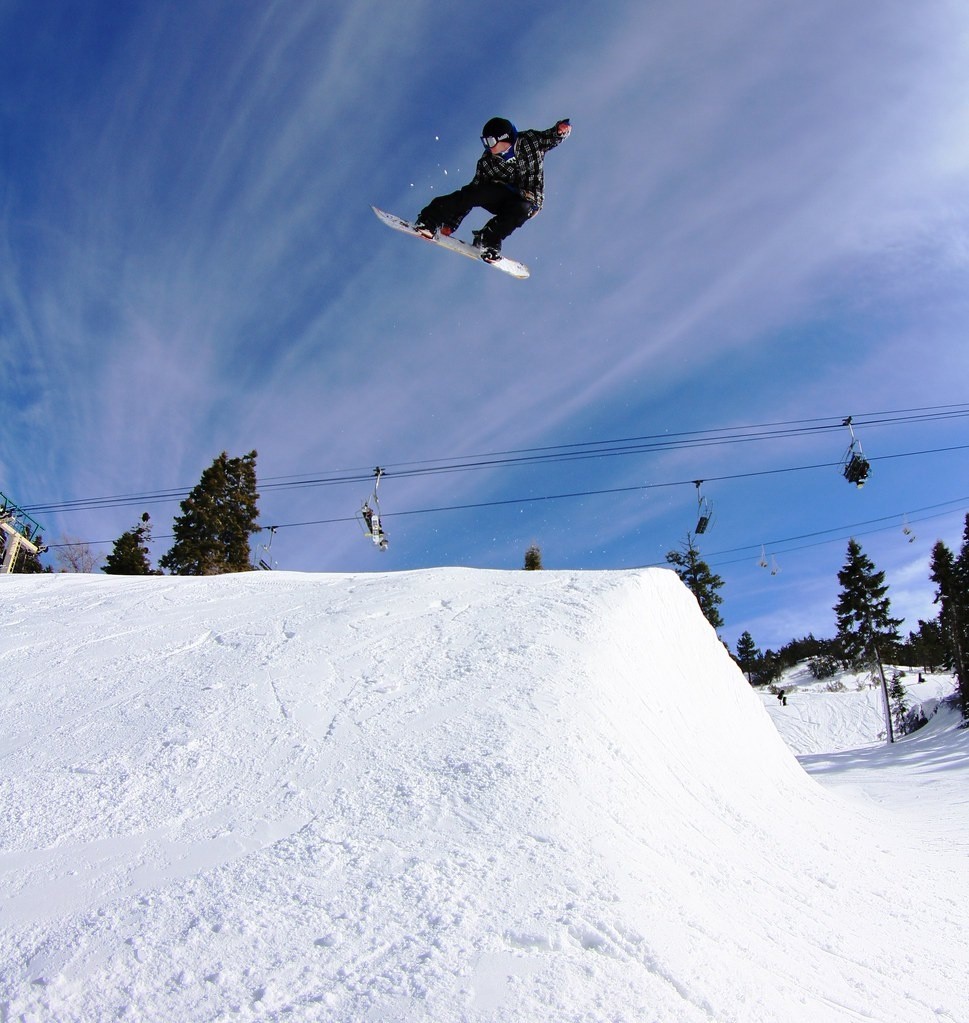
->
[414,117,572,265]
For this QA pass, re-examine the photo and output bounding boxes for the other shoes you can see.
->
[473,229,502,262]
[415,215,440,239]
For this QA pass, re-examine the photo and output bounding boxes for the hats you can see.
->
[482,117,515,143]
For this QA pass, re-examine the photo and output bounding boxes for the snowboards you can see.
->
[368,204,530,281]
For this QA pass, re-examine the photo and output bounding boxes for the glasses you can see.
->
[480,136,498,148]
